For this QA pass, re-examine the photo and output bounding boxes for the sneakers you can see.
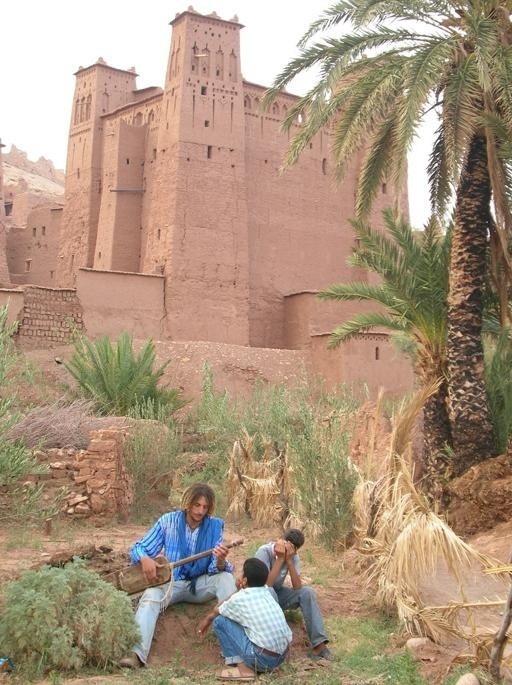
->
[120,652,145,669]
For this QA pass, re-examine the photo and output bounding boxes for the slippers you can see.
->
[307,648,339,662]
[215,667,255,681]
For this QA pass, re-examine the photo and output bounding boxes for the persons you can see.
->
[120,483,244,668]
[196,558,293,682]
[241,528,342,665]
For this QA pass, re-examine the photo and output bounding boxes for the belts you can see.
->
[255,646,289,657]
